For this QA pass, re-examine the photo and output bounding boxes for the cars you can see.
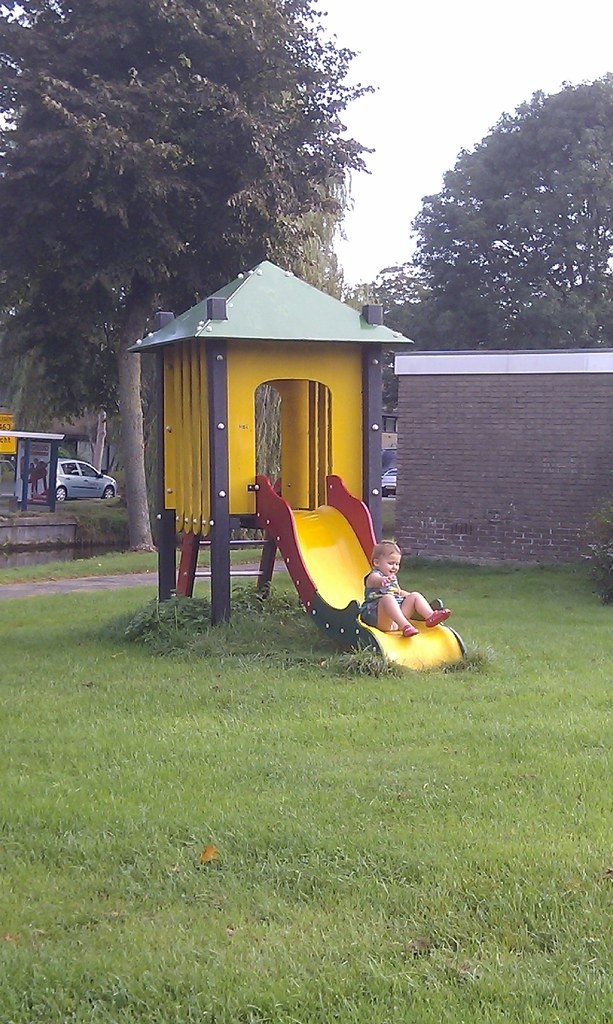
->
[56,457,117,502]
[381,469,397,497]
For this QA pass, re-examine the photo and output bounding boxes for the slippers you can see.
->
[401,624,420,638]
[425,608,452,628]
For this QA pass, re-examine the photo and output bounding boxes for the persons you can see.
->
[360,540,451,637]
[20,455,47,495]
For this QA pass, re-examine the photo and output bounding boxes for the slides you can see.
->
[255,474,467,670]
